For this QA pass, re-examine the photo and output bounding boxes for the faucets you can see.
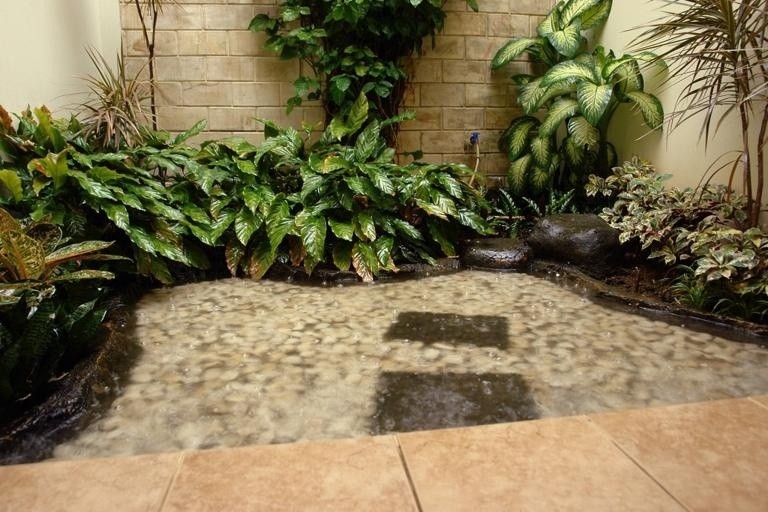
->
[470,131,481,145]
